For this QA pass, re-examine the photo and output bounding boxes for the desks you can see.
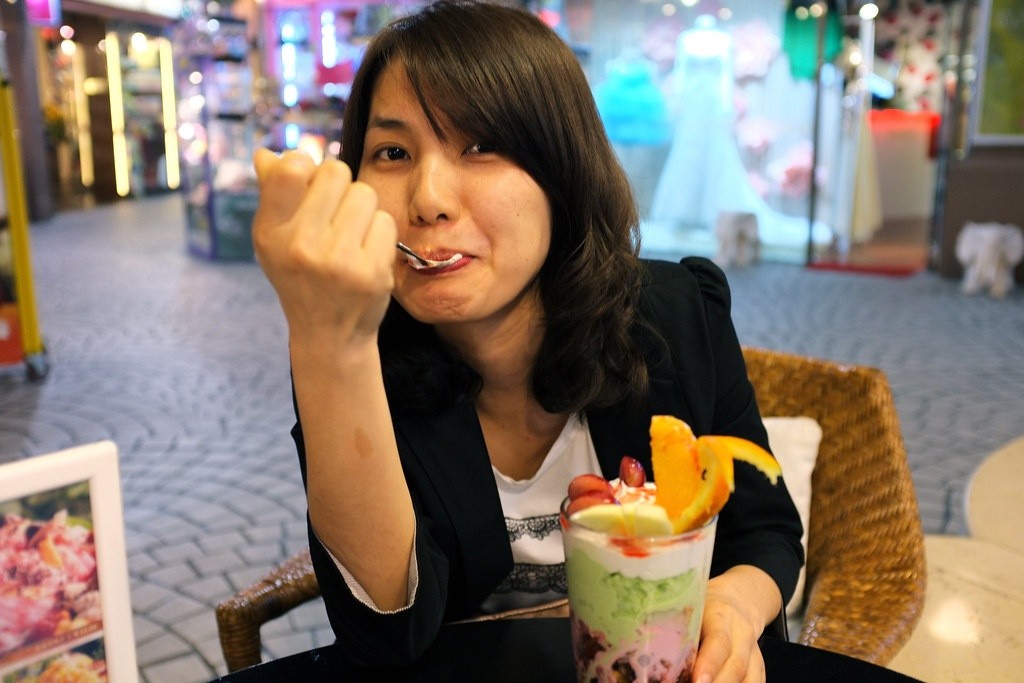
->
[202,617,926,683]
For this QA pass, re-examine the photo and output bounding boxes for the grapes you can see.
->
[567,456,645,517]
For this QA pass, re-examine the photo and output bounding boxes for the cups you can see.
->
[559,481,718,683]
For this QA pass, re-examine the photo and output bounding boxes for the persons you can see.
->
[648,15,755,226]
[252,0,805,683]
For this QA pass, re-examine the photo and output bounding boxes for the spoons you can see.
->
[394,238,453,270]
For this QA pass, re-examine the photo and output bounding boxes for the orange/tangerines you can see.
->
[649,415,780,533]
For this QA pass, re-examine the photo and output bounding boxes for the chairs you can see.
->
[213,345,927,666]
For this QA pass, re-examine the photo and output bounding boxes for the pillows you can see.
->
[761,417,823,619]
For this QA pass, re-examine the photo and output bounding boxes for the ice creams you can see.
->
[556,479,718,683]
